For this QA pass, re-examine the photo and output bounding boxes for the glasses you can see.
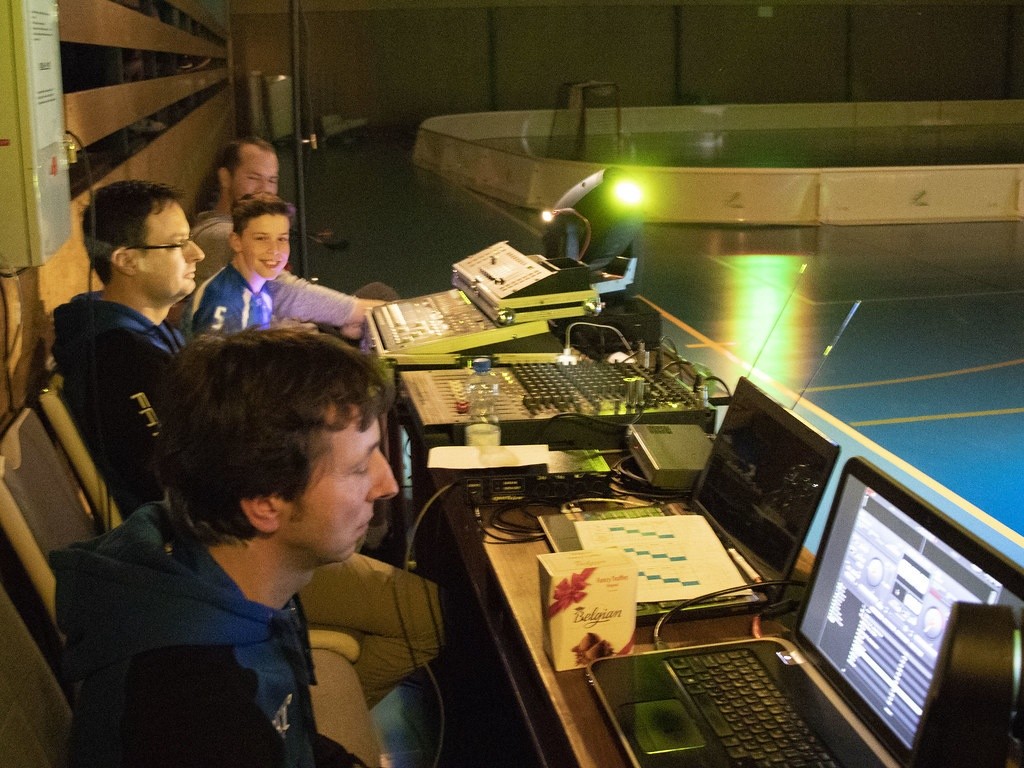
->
[125,233,194,252]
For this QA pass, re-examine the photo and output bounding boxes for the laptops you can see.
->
[534,372,1024,768]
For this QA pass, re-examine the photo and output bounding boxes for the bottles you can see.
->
[462,358,501,447]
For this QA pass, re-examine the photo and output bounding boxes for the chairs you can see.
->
[0,400,103,715]
[40,352,124,535]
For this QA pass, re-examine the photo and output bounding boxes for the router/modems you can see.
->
[624,423,716,487]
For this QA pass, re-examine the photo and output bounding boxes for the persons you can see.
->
[184,135,400,340]
[177,192,297,347]
[50,320,401,767]
[52,181,463,711]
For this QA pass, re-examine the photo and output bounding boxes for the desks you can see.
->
[364,285,1022,768]
